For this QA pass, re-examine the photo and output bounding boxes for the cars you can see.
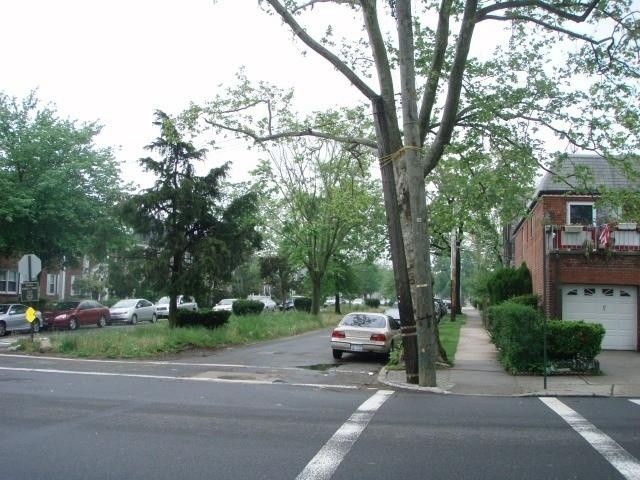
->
[0,294,198,336]
[323,297,363,306]
[331,312,400,359]
[212,295,305,311]
[433,297,451,322]
